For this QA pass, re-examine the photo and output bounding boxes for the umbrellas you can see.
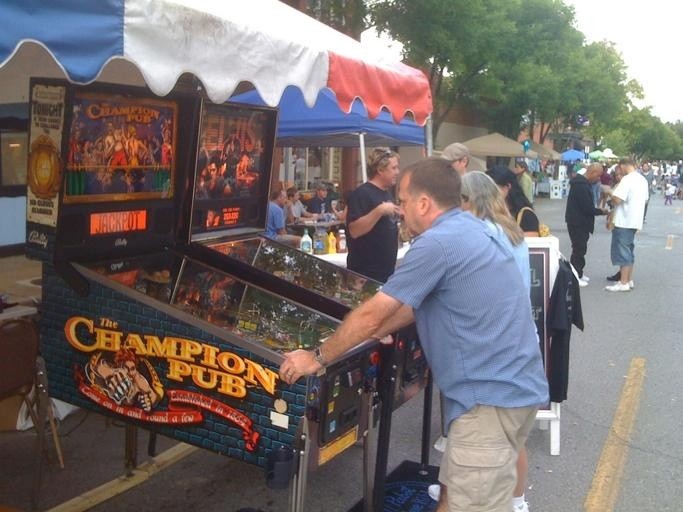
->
[458,132,620,162]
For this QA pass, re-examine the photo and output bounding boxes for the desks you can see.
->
[287,218,345,233]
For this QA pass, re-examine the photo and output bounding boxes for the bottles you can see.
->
[300,227,349,254]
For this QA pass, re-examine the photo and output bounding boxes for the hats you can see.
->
[440,142,471,161]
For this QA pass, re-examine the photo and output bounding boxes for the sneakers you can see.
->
[605,280,635,291]
[579,275,590,287]
[607,271,621,281]
[427,483,441,503]
[513,501,530,512]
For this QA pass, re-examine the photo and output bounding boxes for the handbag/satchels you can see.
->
[516,206,551,237]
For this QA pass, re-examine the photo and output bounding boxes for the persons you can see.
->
[202,157,232,198]
[516,161,533,206]
[257,183,347,249]
[428,171,539,512]
[95,349,160,414]
[72,120,173,186]
[542,156,682,292]
[485,167,551,237]
[345,146,403,283]
[278,156,550,512]
[441,142,470,176]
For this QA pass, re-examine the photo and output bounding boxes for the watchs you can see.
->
[313,349,328,367]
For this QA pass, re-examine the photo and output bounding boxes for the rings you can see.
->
[288,370,293,376]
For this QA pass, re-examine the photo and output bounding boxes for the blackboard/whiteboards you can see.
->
[525,237,560,420]
